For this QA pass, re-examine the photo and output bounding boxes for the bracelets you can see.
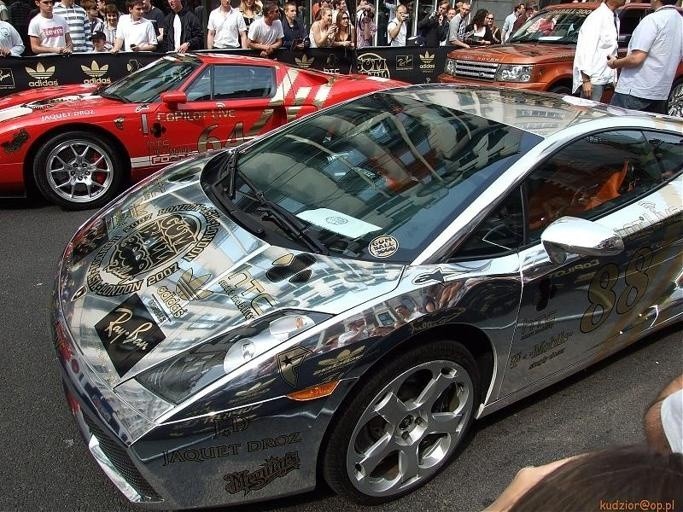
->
[612,60,617,67]
[583,79,590,84]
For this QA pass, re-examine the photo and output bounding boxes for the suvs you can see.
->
[436,2,683,119]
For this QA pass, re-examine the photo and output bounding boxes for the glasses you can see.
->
[342,17,350,20]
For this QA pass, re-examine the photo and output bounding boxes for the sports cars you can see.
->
[0,51,412,211]
[48,80,683,512]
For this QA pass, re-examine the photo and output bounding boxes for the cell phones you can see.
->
[130,44,136,51]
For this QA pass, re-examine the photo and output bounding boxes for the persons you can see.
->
[643,374,683,455]
[607,0,683,116]
[482,443,683,511]
[0,0,538,66]
[569,0,626,103]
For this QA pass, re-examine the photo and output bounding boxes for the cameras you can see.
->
[402,14,410,21]
[435,12,441,19]
[365,9,374,18]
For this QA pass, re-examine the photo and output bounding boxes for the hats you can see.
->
[89,32,106,41]
